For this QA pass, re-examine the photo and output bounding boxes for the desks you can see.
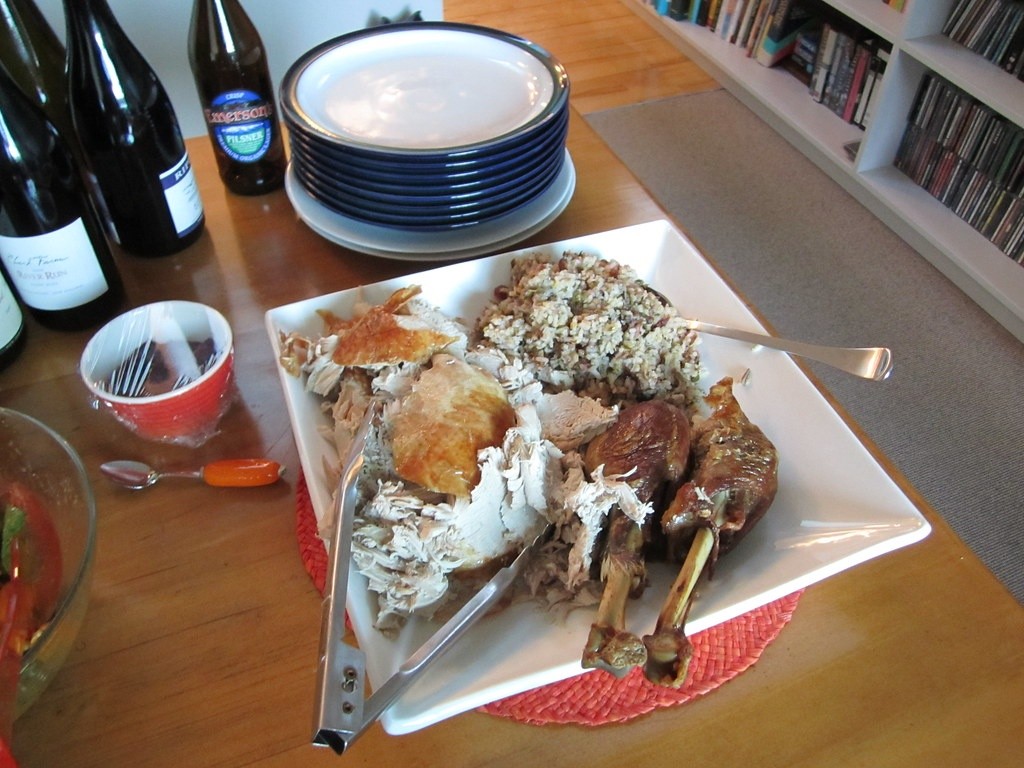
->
[0,102,1024,768]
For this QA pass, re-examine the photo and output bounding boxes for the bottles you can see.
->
[187,0,288,196]
[0,0,207,370]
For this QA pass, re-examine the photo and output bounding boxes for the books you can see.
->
[893,67,1024,272]
[943,0,1024,80]
[648,0,905,131]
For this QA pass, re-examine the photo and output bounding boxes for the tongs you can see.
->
[310,398,557,756]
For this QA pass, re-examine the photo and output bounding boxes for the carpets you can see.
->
[583,84,1024,616]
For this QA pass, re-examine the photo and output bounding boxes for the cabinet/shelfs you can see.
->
[625,0,1024,344]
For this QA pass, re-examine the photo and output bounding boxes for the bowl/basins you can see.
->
[78,299,234,444]
[0,406,98,721]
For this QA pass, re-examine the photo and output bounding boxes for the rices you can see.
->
[467,248,703,413]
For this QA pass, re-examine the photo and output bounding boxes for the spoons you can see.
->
[100,459,284,490]
[643,287,893,383]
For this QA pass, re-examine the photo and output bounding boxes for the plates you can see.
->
[264,219,932,739]
[277,21,578,262]
